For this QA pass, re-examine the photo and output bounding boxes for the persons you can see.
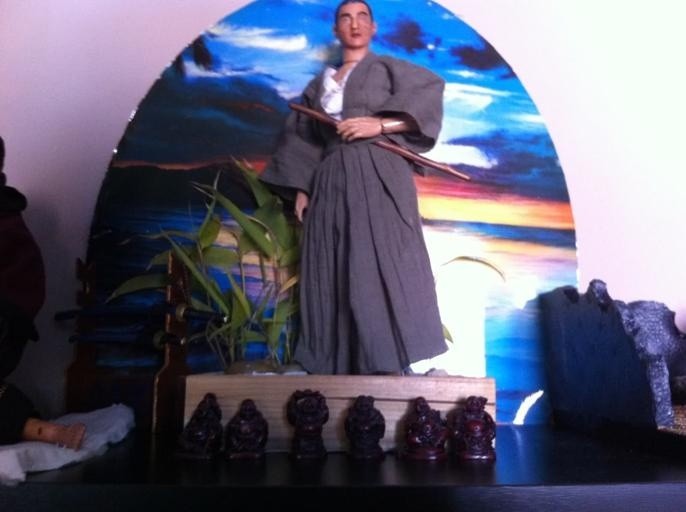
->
[183,390,222,450]
[0,139,87,453]
[444,394,498,466]
[282,387,331,465]
[343,394,386,466]
[223,396,270,455]
[257,1,451,381]
[398,396,452,467]
